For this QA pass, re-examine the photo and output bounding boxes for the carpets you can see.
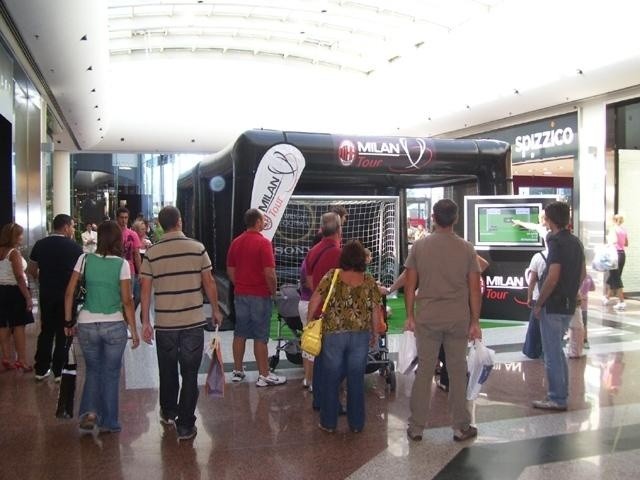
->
[269,292,525,341]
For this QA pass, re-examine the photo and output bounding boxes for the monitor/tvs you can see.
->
[474,204,544,246]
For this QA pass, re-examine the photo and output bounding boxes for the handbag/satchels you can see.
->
[465,339,495,400]
[206,324,225,398]
[73,279,88,314]
[56,344,77,418]
[301,318,322,356]
[523,311,542,358]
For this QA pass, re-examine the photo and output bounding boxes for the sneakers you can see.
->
[35,368,51,380]
[79,414,121,432]
[160,408,197,440]
[256,371,287,386]
[232,366,246,380]
[407,428,422,441]
[437,380,448,391]
[533,398,566,409]
[613,301,625,309]
[453,426,477,440]
[303,378,313,392]
[600,295,608,305]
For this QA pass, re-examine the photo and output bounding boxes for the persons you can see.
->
[1,223,35,374]
[28,214,82,383]
[65,219,139,432]
[0,198,596,443]
[524,200,596,411]
[379,198,489,442]
[80,207,154,338]
[297,204,388,434]
[225,208,287,387]
[600,214,629,310]
[138,204,224,439]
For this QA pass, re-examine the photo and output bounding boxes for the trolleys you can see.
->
[368,296,396,393]
[269,283,302,372]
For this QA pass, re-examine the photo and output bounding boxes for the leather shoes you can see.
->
[312,406,363,432]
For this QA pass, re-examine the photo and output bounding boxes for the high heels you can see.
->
[3,359,32,371]
